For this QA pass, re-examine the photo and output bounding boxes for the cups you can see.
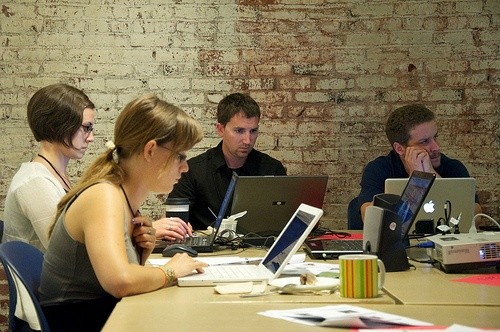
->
[163,198,192,226]
[339,254,386,299]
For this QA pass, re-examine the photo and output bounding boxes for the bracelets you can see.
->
[159,265,175,288]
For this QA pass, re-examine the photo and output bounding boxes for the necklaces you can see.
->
[38,154,71,191]
[119,183,137,217]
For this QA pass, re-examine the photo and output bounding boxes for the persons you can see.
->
[167,93,287,231]
[358,105,483,225]
[2,84,96,254]
[37,93,210,332]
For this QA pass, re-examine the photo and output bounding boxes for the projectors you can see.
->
[426,231,500,273]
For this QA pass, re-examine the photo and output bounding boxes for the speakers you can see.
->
[363,206,409,271]
[373,193,400,211]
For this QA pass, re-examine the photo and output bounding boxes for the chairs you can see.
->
[0,241,51,332]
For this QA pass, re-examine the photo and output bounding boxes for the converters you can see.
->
[242,236,274,247]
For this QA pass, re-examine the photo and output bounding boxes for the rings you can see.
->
[149,227,152,233]
[169,226,172,230]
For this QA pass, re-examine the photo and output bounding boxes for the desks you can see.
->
[100,229,500,332]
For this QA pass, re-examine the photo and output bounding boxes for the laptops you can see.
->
[229,176,330,236]
[303,170,437,259]
[177,203,324,286]
[151,171,238,253]
[384,177,476,238]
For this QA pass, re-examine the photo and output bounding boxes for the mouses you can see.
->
[162,244,198,257]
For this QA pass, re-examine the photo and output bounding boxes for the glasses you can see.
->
[156,144,187,164]
[81,124,93,132]
